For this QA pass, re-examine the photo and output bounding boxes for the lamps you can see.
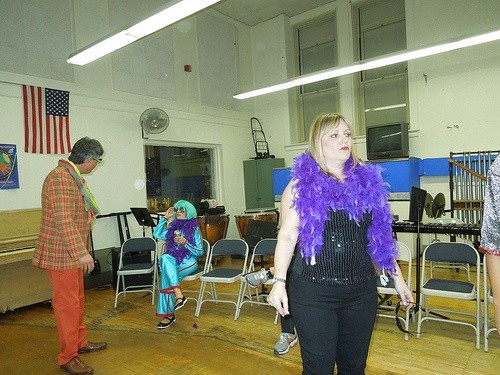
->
[233,28,500,101]
[66,0,221,64]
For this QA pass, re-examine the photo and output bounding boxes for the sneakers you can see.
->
[245,268,275,288]
[274,328,298,354]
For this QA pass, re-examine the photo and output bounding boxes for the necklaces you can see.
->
[291,148,398,285]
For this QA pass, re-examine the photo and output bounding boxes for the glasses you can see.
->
[395,300,422,336]
[174,207,185,213]
[92,157,103,166]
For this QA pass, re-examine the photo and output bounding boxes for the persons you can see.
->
[32,138,108,375]
[153,200,204,328]
[245,267,298,355]
[266,112,414,375]
[479,153,500,337]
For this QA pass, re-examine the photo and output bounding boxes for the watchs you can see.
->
[273,277,287,283]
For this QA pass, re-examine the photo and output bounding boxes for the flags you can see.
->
[22,85,73,155]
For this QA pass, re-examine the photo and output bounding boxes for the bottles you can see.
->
[148,196,172,213]
[174,229,180,243]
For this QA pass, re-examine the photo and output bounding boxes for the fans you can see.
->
[139,107,170,134]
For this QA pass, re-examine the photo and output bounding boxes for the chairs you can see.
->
[113,237,495,352]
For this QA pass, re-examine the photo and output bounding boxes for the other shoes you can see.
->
[157,315,176,328]
[174,295,187,311]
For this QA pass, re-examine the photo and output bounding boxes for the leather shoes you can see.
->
[60,356,94,375]
[78,341,107,355]
